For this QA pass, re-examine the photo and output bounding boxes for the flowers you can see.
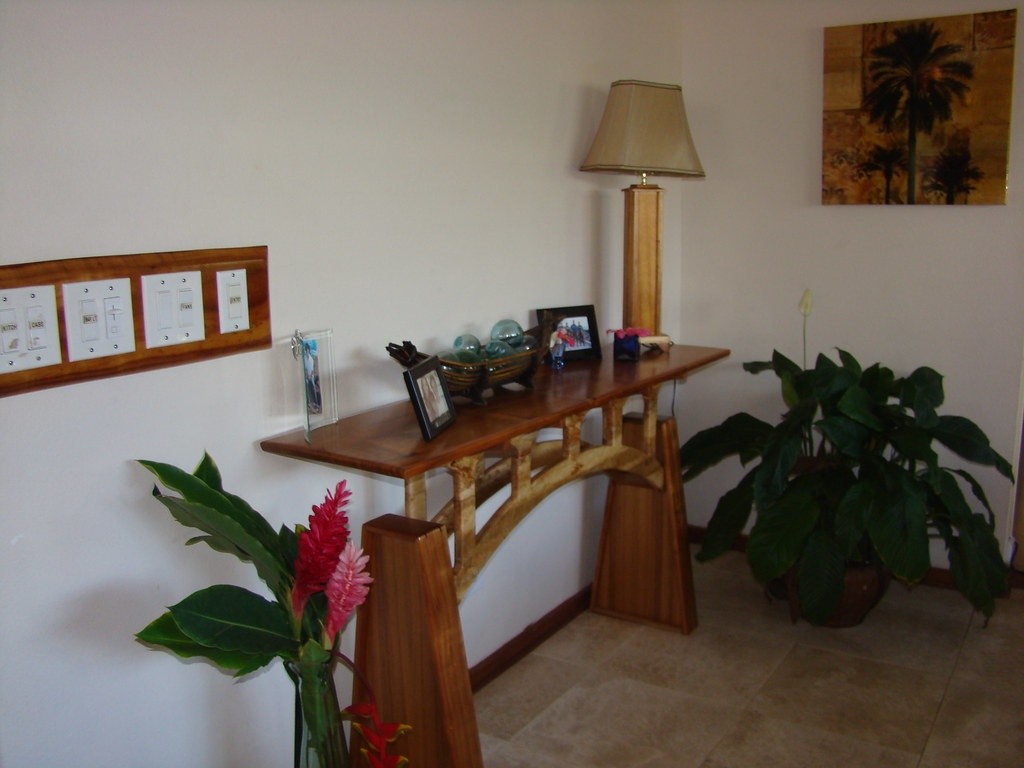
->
[679,284,1016,631]
[135,448,413,767]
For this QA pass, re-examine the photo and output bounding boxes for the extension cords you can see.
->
[639,334,670,345]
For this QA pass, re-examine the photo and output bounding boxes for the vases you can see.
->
[786,565,885,630]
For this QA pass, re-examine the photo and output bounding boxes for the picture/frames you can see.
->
[403,354,457,442]
[535,304,602,363]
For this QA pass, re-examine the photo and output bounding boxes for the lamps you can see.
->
[580,79,709,336]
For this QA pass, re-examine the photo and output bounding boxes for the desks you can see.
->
[261,338,732,768]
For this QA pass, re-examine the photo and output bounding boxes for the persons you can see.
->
[558,318,585,348]
[420,376,438,419]
[303,342,318,414]
[429,375,449,418]
